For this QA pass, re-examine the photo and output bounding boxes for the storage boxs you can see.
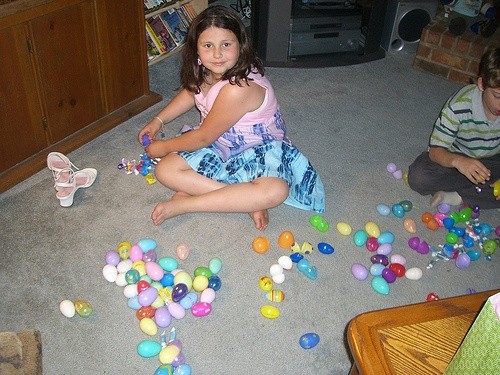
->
[444,292,500,375]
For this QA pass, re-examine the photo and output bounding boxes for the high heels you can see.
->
[56,167,97,207]
[46,151,80,192]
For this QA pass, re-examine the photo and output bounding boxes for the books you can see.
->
[143,0,199,63]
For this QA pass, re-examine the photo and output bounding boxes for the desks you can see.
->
[343,289,500,375]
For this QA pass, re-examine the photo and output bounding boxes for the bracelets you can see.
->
[154,116,165,134]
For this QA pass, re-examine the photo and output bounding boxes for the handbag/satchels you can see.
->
[442,292,500,375]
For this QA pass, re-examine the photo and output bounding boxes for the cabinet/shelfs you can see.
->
[0,0,163,195]
[143,0,208,66]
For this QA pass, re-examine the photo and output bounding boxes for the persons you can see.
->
[138,5,325,232]
[408,48,500,210]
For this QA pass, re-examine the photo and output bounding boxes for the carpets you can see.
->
[0,329,43,375]
